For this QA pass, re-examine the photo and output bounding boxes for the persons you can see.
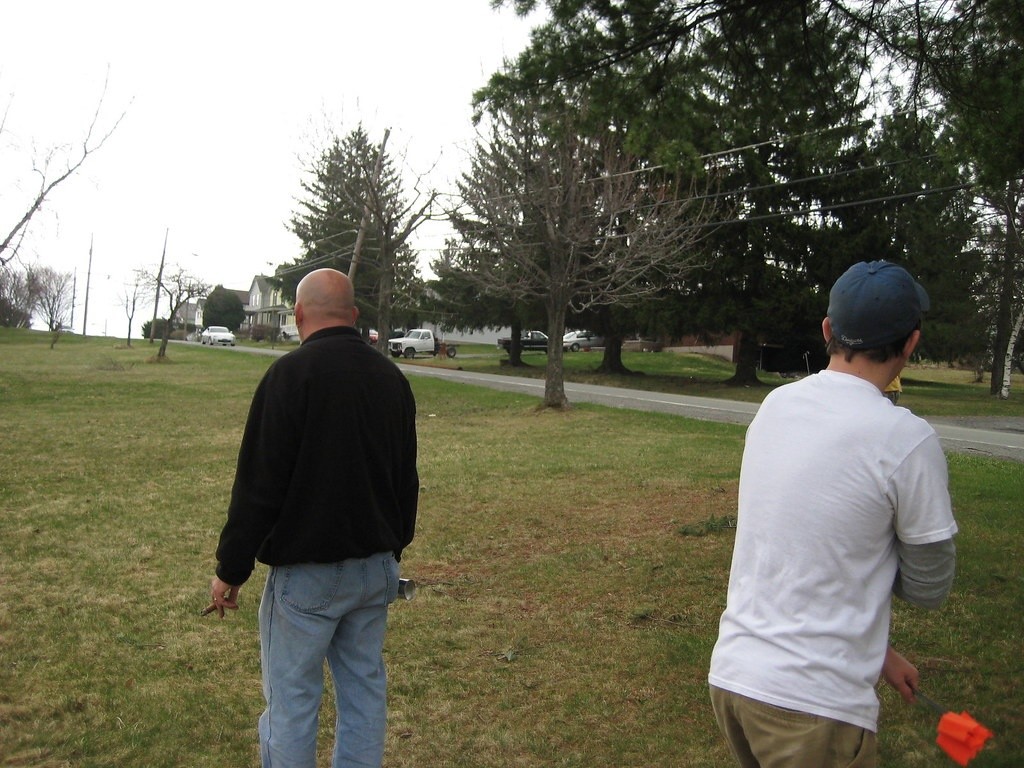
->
[212,268,419,768]
[881,376,902,405]
[707,264,959,768]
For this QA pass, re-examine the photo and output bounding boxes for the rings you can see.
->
[214,597,217,601]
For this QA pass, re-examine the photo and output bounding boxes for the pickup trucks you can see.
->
[497,332,550,353]
[388,330,455,361]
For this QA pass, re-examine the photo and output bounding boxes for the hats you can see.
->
[827,260,930,350]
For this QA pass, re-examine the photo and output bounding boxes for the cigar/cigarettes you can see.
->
[200,596,228,616]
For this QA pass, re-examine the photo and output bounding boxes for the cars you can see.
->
[361,329,380,345]
[200,326,235,346]
[562,330,606,353]
